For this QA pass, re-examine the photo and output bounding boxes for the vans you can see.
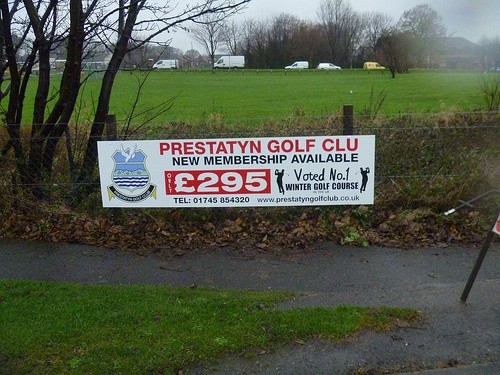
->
[152,59,179,70]
[285,61,309,69]
[214,55,245,71]
[318,63,342,70]
[363,62,386,69]
